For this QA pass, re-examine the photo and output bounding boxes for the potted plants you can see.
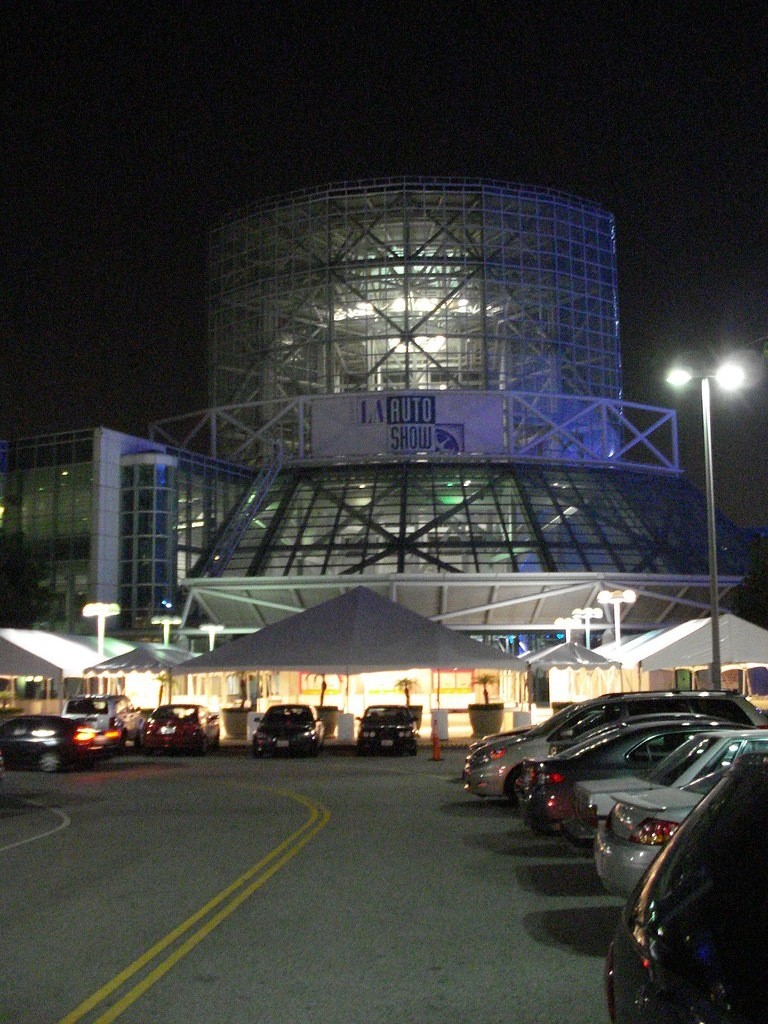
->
[306,673,343,735]
[140,673,179,721]
[222,670,256,736]
[468,672,504,736]
[393,678,423,730]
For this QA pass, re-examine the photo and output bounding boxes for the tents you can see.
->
[593,613,768,672]
[523,641,624,693]
[178,586,528,675]
[0,627,140,711]
[84,643,194,702]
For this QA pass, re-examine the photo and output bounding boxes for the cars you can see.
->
[355,705,421,756]
[602,751,768,1024]
[252,704,325,758]
[594,730,768,897]
[565,729,757,846]
[514,713,759,835]
[60,693,155,756]
[0,713,107,771]
[140,704,221,758]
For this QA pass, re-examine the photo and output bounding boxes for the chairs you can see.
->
[396,712,404,717]
[371,712,377,716]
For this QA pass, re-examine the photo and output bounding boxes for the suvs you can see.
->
[464,689,768,807]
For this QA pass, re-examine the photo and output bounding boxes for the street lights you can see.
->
[597,589,636,664]
[571,607,602,653]
[199,623,225,652]
[555,617,582,645]
[665,362,748,692]
[151,615,183,645]
[82,602,121,662]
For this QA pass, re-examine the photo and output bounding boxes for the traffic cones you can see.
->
[429,720,445,761]
